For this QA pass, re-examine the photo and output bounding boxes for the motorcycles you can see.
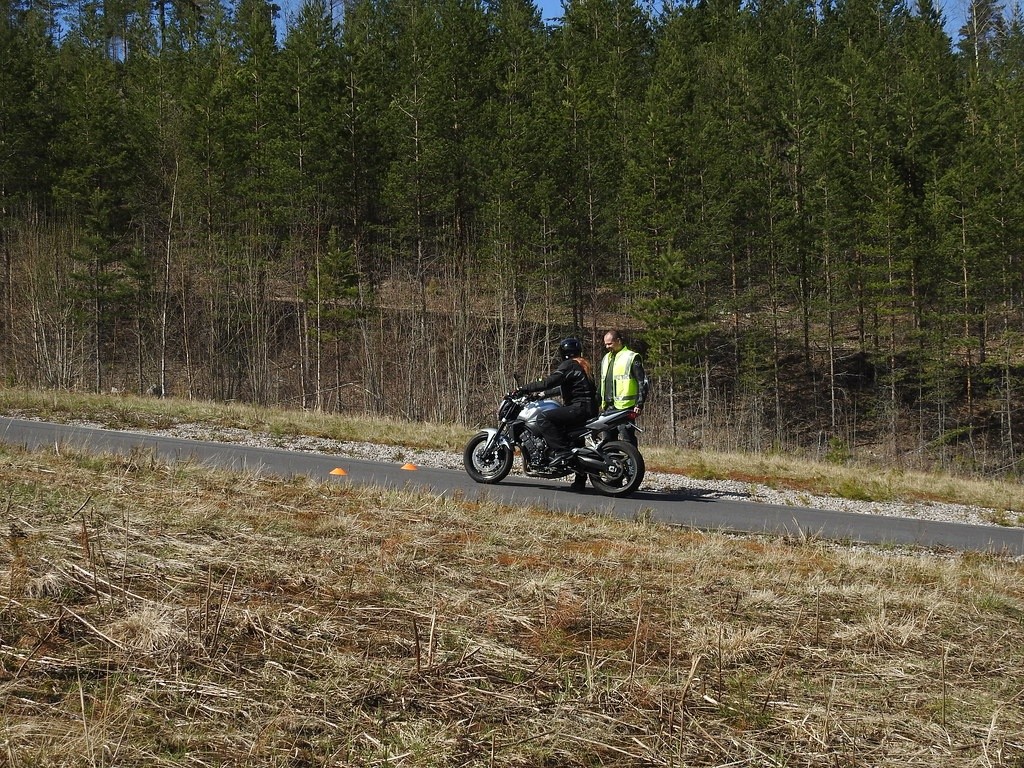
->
[463,372,645,497]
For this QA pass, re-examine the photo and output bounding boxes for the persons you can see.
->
[503,338,602,490]
[597,330,650,491]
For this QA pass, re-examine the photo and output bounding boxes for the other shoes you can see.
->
[567,482,586,493]
[548,450,573,467]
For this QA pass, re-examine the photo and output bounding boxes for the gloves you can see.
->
[508,388,523,399]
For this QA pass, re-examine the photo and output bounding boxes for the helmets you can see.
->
[559,338,582,361]
